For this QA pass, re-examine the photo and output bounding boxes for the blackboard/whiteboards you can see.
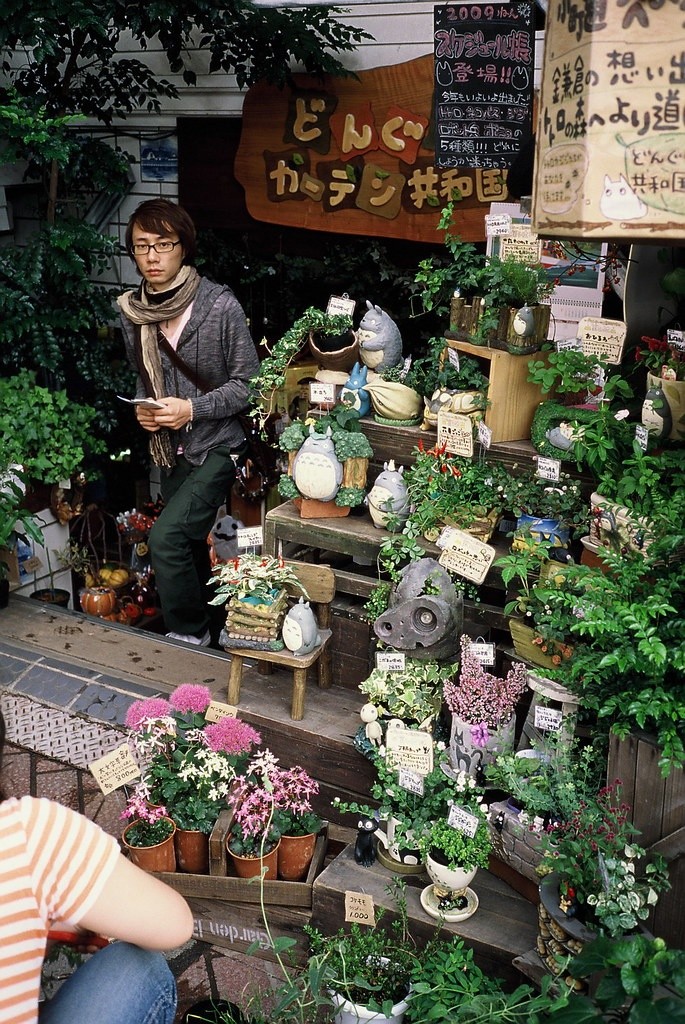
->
[432,3,537,196]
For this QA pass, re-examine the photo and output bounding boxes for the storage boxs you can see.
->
[255,358,320,424]
[0,504,76,613]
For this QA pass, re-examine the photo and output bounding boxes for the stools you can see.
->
[514,669,601,770]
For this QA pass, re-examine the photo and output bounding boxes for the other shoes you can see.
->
[165,628,211,648]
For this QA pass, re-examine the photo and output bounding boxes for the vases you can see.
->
[450,713,516,784]
[532,869,656,998]
[515,513,574,562]
[225,832,282,881]
[78,585,117,618]
[507,616,590,681]
[122,814,176,874]
[424,852,480,892]
[143,788,170,817]
[372,811,439,866]
[277,832,316,882]
[29,586,70,611]
[174,827,211,874]
[646,370,685,443]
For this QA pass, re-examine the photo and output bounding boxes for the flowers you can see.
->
[514,551,595,666]
[518,777,631,887]
[499,453,580,518]
[117,680,318,837]
[441,636,528,747]
[633,335,685,383]
[328,739,503,849]
[114,493,166,545]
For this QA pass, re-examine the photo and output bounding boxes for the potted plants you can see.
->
[0,468,45,610]
[201,551,312,643]
[363,336,491,436]
[524,350,637,464]
[288,873,458,1024]
[246,304,359,445]
[275,392,372,508]
[654,247,685,353]
[0,368,107,504]
[405,199,556,356]
[400,435,501,547]
[585,438,685,567]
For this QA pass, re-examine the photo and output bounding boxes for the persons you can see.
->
[117,198,260,646]
[0,713,193,1024]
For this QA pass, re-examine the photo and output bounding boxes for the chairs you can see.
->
[227,560,336,719]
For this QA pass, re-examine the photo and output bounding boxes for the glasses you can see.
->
[130,240,181,255]
